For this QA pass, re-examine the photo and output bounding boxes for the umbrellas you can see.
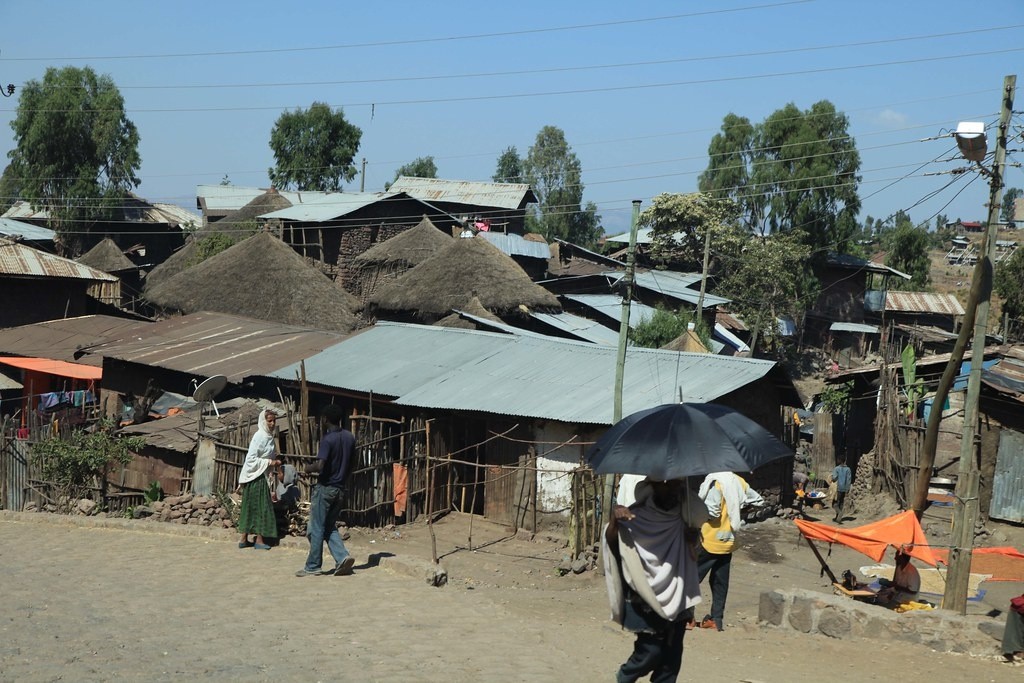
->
[587,386,795,523]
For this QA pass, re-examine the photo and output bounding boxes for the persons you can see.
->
[271,464,295,520]
[685,472,764,629]
[238,410,281,549]
[296,407,356,577]
[602,473,709,683]
[793,471,808,499]
[617,475,648,507]
[832,455,851,523]
[873,550,921,610]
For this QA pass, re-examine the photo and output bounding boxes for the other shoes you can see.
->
[333,556,355,575]
[254,542,270,549]
[686,618,696,630]
[836,512,843,524]
[700,617,718,631]
[297,568,323,577]
[240,542,250,548]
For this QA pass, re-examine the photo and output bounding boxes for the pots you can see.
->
[800,424,813,443]
[930,477,956,488]
